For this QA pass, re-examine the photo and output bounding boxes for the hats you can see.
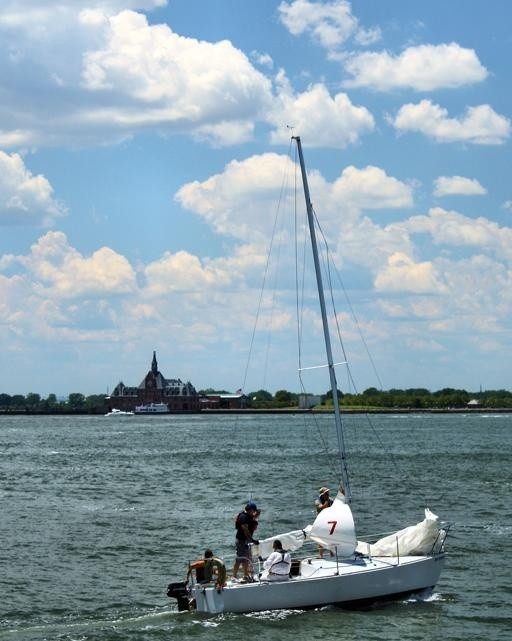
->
[247,502,258,512]
[319,487,330,496]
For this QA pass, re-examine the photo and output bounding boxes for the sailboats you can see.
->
[162,133,454,616]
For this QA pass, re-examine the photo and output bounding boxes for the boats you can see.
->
[132,401,170,414]
[103,407,135,416]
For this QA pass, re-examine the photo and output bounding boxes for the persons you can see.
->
[260,539,293,582]
[230,501,262,583]
[188,550,219,585]
[314,486,335,559]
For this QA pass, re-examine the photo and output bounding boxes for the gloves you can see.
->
[251,539,259,545]
